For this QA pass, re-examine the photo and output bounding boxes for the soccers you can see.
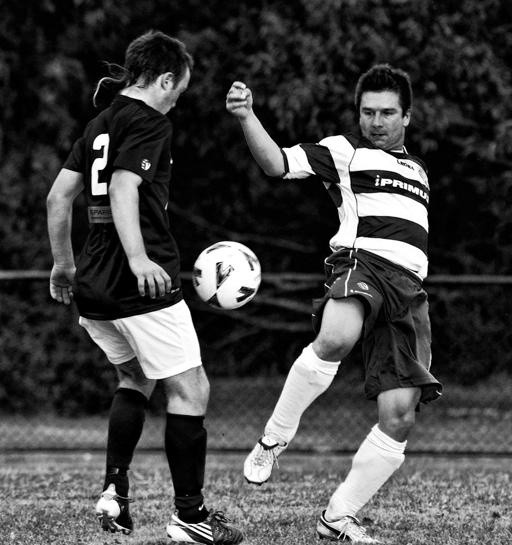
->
[192,242,262,312]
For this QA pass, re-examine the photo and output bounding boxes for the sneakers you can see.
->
[243,433,289,485]
[315,509,381,544]
[95,483,134,535]
[165,509,245,544]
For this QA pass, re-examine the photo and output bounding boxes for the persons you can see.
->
[225,62,444,544]
[45,28,244,544]
